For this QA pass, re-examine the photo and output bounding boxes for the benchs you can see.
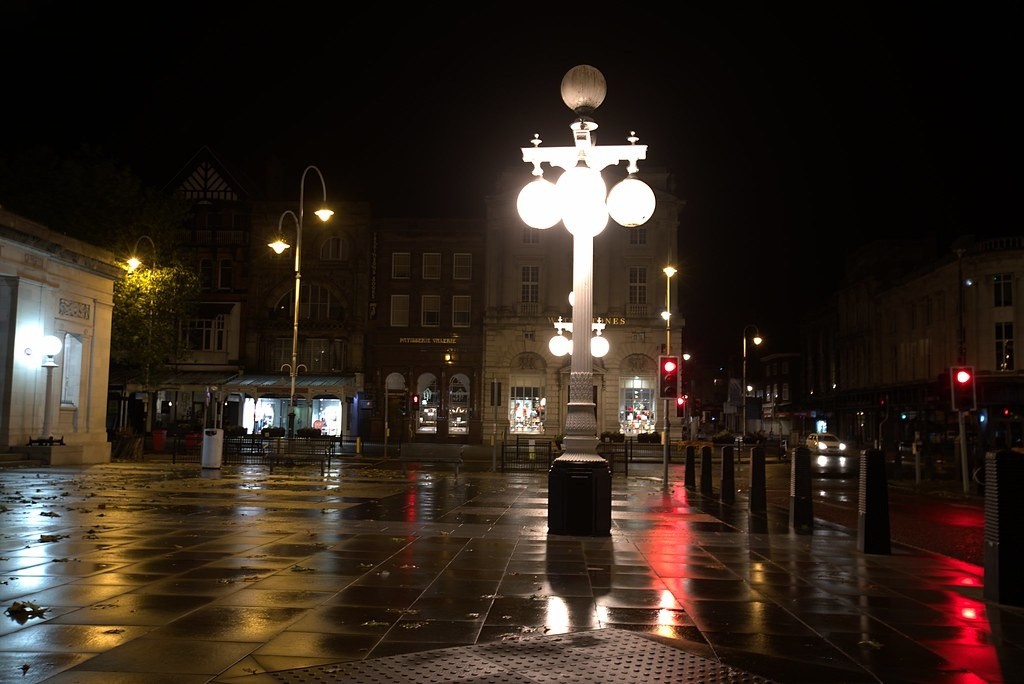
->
[264,438,332,476]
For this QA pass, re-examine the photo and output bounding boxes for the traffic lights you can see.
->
[660,355,680,400]
[676,397,685,418]
[411,393,421,411]
[947,365,977,413]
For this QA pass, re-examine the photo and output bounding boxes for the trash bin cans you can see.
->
[202,428,224,469]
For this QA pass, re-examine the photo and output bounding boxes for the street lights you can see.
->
[516,66,658,537]
[128,234,159,457]
[41,336,62,442]
[740,323,763,438]
[270,164,336,454]
[661,250,678,356]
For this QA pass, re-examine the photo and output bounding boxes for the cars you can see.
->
[808,432,847,455]
